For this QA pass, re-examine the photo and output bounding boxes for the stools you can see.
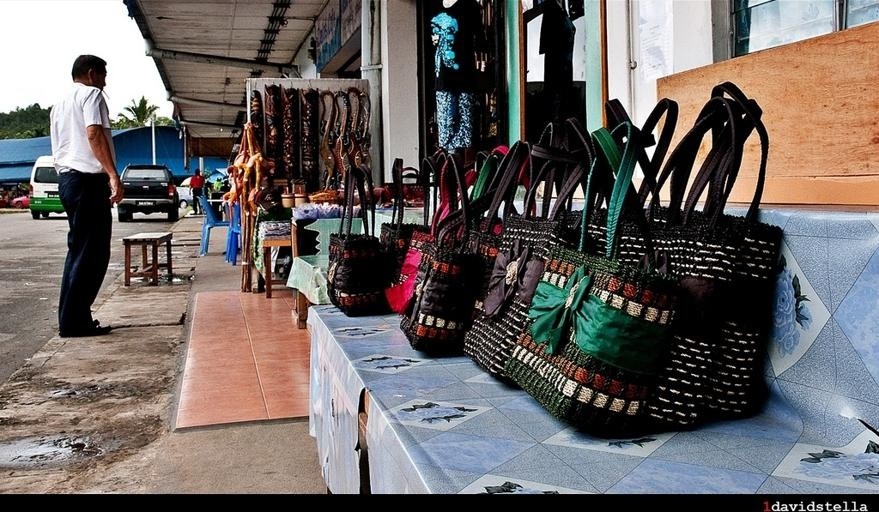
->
[118,231,175,289]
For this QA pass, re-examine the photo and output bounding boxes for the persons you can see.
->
[49,55,125,338]
[189,169,230,215]
[102,90,110,99]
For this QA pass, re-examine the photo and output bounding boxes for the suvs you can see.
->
[116,163,179,224]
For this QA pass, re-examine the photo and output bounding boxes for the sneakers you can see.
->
[190,212,202,215]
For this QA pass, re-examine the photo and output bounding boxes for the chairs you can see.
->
[194,186,244,268]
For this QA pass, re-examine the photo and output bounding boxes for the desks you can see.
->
[261,236,293,299]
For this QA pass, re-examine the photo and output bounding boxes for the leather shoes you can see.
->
[59,320,111,337]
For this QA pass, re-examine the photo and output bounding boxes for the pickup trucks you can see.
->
[175,185,196,210]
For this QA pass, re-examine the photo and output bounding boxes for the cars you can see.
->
[10,194,30,210]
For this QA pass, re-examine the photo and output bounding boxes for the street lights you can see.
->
[149,110,158,165]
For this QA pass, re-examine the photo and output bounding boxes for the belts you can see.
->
[60,168,77,174]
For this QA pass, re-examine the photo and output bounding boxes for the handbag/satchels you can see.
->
[326,82,782,440]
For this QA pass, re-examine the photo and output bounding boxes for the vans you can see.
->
[28,155,67,220]
